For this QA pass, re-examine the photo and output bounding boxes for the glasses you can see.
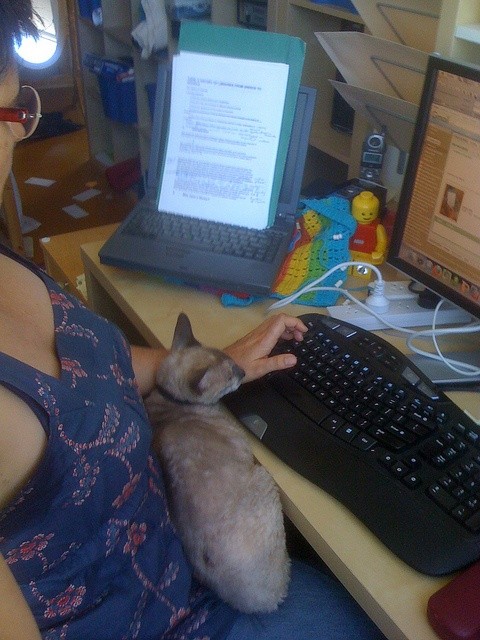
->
[1,85,43,140]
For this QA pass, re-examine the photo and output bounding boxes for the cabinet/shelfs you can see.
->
[40,221,122,311]
[73,0,480,209]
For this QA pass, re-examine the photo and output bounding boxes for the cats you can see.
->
[144,312,291,614]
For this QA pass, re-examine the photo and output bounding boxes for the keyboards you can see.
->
[223,311,479,575]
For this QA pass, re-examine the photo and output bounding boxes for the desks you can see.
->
[80,239,475,637]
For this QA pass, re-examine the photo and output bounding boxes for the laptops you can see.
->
[97,60,317,293]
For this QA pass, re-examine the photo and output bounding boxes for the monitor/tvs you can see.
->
[384,55,480,392]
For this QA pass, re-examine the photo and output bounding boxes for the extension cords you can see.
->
[367,279,422,299]
[326,297,470,329]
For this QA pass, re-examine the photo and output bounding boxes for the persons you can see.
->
[0,0,382,637]
[347,189,388,279]
